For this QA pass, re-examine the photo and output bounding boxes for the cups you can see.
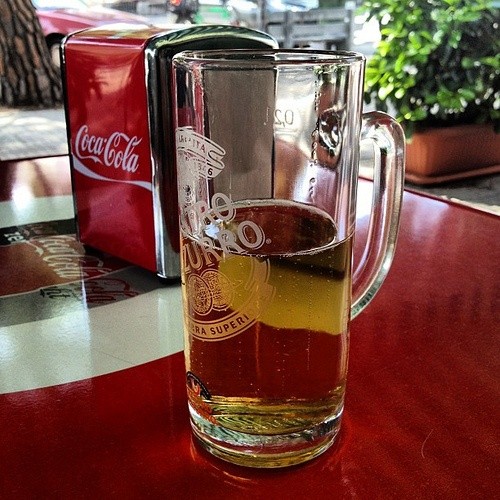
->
[173,49,406,470]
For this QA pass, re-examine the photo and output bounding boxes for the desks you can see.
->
[1,109,499,500]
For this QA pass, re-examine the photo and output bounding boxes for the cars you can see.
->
[32,0,149,68]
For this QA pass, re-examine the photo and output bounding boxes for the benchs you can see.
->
[258,0,354,53]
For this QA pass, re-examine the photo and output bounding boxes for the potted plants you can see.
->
[358,0,499,186]
